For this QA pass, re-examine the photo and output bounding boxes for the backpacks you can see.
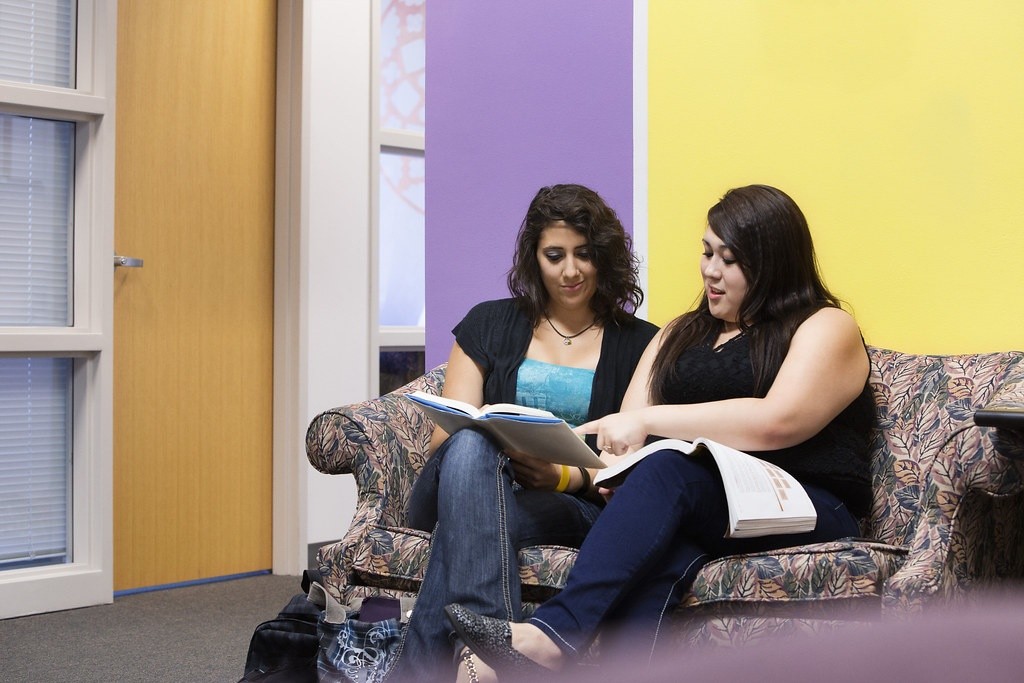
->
[237,594,319,683]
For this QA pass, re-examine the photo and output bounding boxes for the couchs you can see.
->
[304,346,1024,658]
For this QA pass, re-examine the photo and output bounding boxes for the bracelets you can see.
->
[554,465,570,492]
[567,467,590,497]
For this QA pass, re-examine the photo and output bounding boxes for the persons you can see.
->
[381,184,661,682]
[446,185,876,683]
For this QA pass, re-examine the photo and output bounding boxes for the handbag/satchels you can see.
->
[308,581,417,683]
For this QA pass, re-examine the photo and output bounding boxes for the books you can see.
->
[593,436,817,539]
[403,390,608,469]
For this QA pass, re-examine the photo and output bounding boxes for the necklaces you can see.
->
[712,321,755,351]
[543,309,601,345]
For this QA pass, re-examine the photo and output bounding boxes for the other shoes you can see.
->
[455,648,498,683]
[444,603,563,683]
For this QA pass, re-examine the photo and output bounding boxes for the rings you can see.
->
[603,446,611,449]
